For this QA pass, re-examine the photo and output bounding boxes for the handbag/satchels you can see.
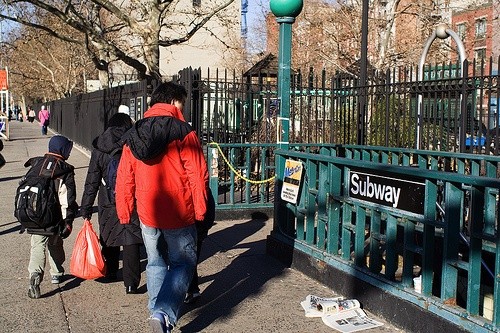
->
[44,119,50,126]
[70,219,108,280]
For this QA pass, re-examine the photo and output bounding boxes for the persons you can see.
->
[80,82,215,333]
[8,105,36,124]
[28,136,78,299]
[39,106,50,135]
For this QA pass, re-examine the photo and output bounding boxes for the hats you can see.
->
[41,105,46,110]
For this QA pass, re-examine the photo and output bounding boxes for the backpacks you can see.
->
[104,158,118,206]
[13,166,70,235]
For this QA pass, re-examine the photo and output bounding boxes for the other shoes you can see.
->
[126,285,137,294]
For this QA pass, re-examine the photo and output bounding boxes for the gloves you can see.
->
[81,208,93,220]
[60,220,73,238]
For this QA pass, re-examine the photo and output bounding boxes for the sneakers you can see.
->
[27,273,43,299]
[184,290,201,305]
[51,275,60,284]
[149,311,170,333]
